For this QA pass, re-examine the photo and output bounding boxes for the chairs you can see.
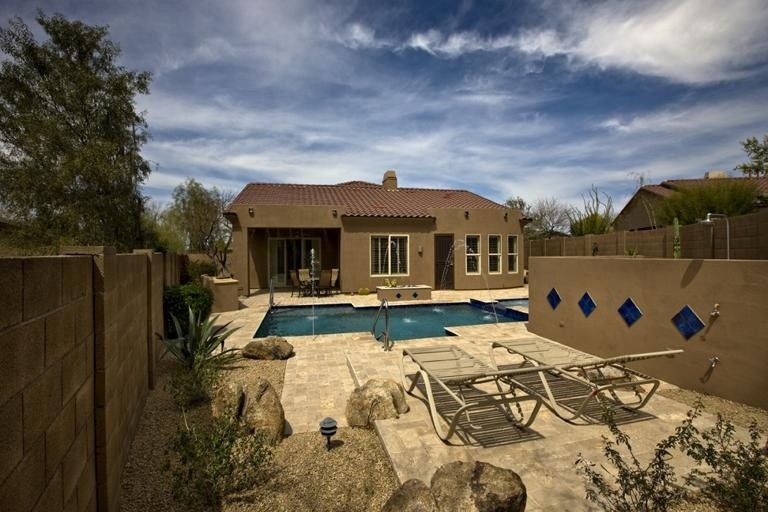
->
[403,337,684,446]
[289,268,340,299]
